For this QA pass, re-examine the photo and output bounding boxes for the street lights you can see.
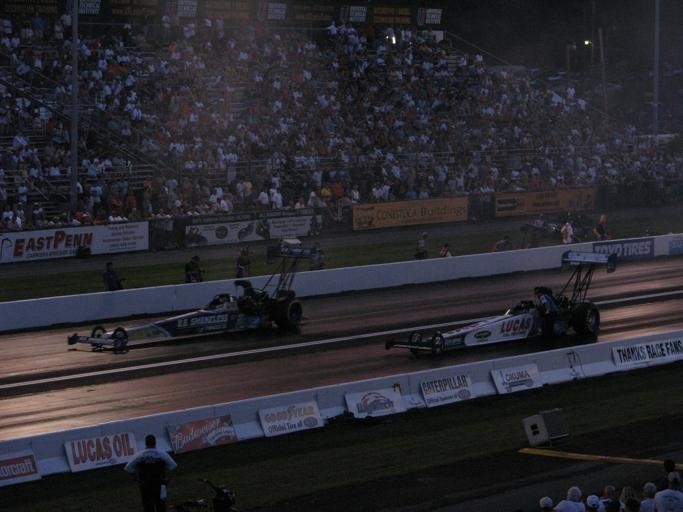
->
[583,40,595,65]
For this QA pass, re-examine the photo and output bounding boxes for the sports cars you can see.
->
[382,250,619,360]
[66,245,326,354]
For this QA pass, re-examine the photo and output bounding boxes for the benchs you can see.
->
[0,0,683,231]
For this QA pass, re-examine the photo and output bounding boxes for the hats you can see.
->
[539,468,683,510]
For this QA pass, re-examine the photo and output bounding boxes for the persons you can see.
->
[101,262,122,291]
[539,457,682,511]
[235,247,254,278]
[184,255,202,283]
[308,241,327,270]
[532,288,559,349]
[121,431,178,511]
[1,2,681,267]
